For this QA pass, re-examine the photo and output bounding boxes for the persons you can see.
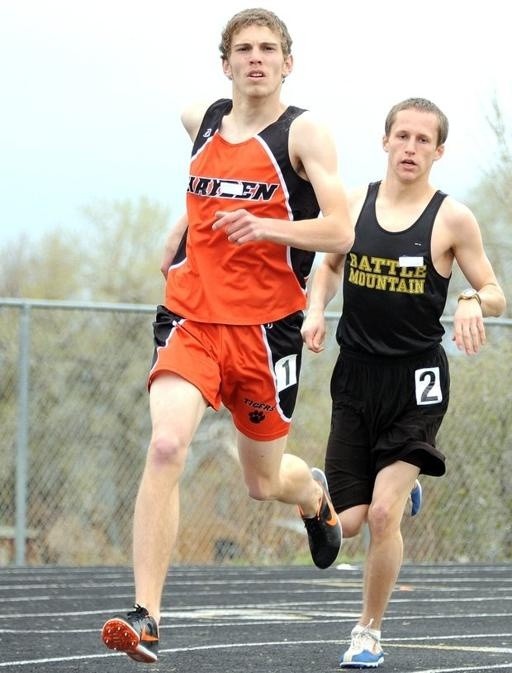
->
[301,98,505,667]
[101,9,355,663]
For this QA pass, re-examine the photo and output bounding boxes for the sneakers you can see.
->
[101,604,160,663]
[403,479,423,517]
[297,468,342,569]
[338,618,385,667]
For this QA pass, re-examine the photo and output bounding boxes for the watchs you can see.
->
[456,286,481,304]
